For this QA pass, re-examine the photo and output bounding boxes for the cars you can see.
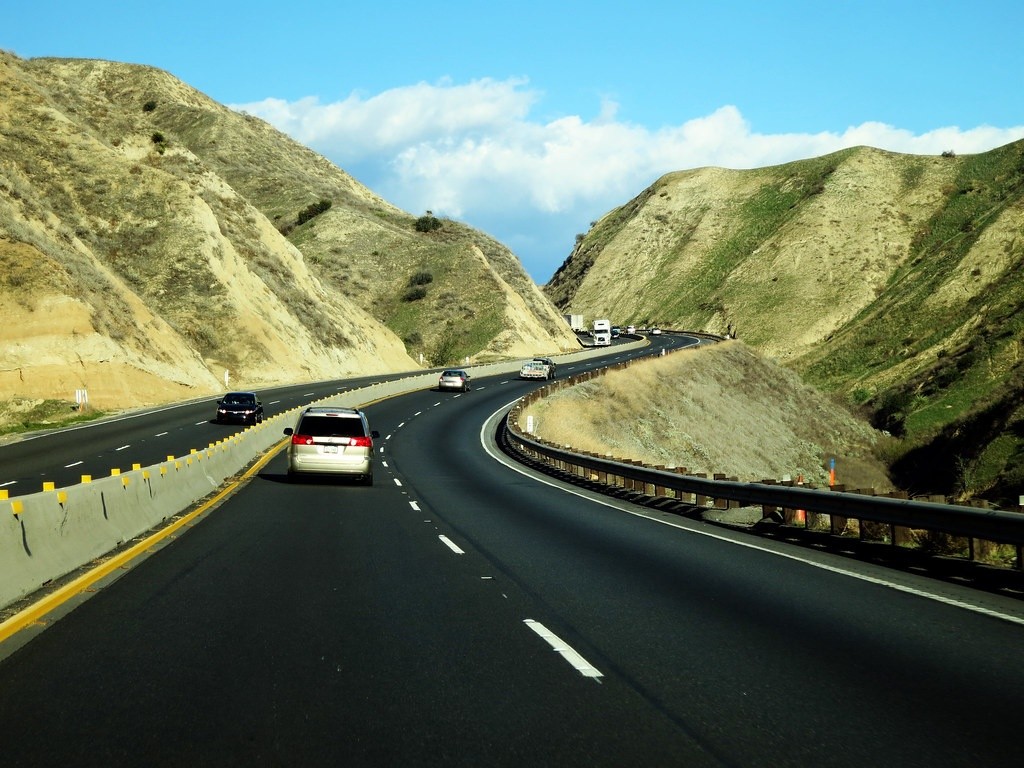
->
[438,369,472,392]
[215,391,264,427]
[647,328,662,336]
[587,325,636,339]
[283,406,380,485]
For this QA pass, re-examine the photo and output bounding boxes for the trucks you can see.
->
[563,313,583,333]
[591,320,612,346]
[520,356,557,381]
